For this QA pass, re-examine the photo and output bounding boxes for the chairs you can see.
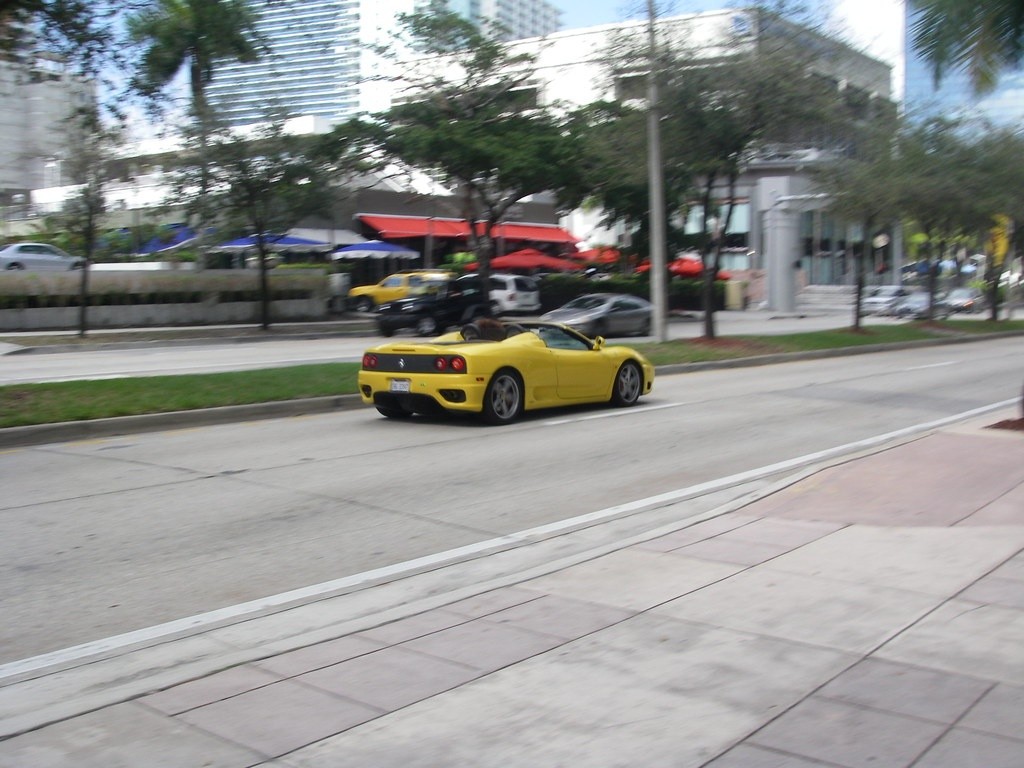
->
[505,324,526,339]
[460,323,484,341]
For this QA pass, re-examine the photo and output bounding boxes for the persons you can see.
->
[479,319,505,340]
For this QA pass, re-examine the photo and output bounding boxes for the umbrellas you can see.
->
[566,245,640,263]
[326,240,421,261]
[89,224,372,258]
[635,258,730,280]
[464,247,584,270]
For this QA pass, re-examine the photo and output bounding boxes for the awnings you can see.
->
[352,213,579,243]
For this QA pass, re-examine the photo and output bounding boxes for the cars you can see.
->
[0,242,89,272]
[536,292,654,339]
[855,256,1023,321]
[344,267,540,338]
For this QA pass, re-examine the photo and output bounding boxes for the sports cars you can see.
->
[355,319,655,427]
